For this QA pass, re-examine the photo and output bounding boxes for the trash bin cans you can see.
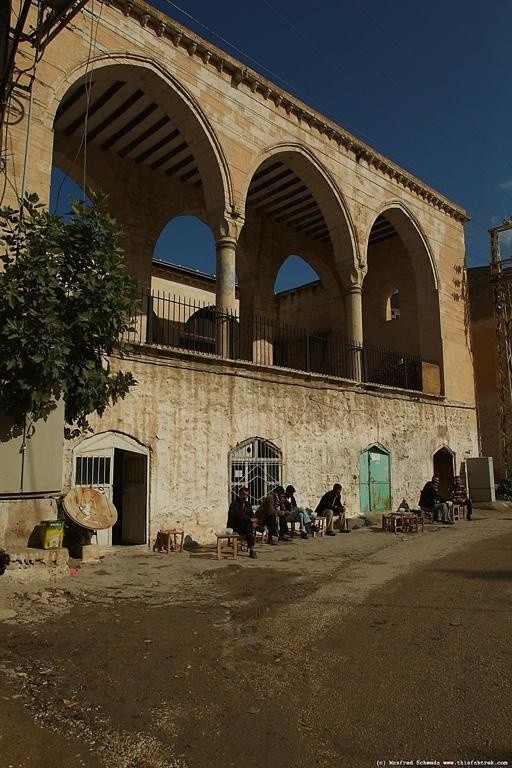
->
[40,520,64,549]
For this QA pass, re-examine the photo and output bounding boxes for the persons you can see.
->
[226,486,266,559]
[418,475,454,525]
[314,483,351,536]
[255,485,320,545]
[448,476,474,520]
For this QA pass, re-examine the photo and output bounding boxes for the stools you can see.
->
[159,528,184,554]
[382,501,466,535]
[214,514,327,561]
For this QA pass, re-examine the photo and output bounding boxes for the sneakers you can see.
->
[249,550,257,559]
[267,538,277,545]
[301,531,308,539]
[340,529,351,533]
[436,513,472,524]
[311,525,320,532]
[255,526,265,533]
[278,535,293,541]
[326,530,337,536]
